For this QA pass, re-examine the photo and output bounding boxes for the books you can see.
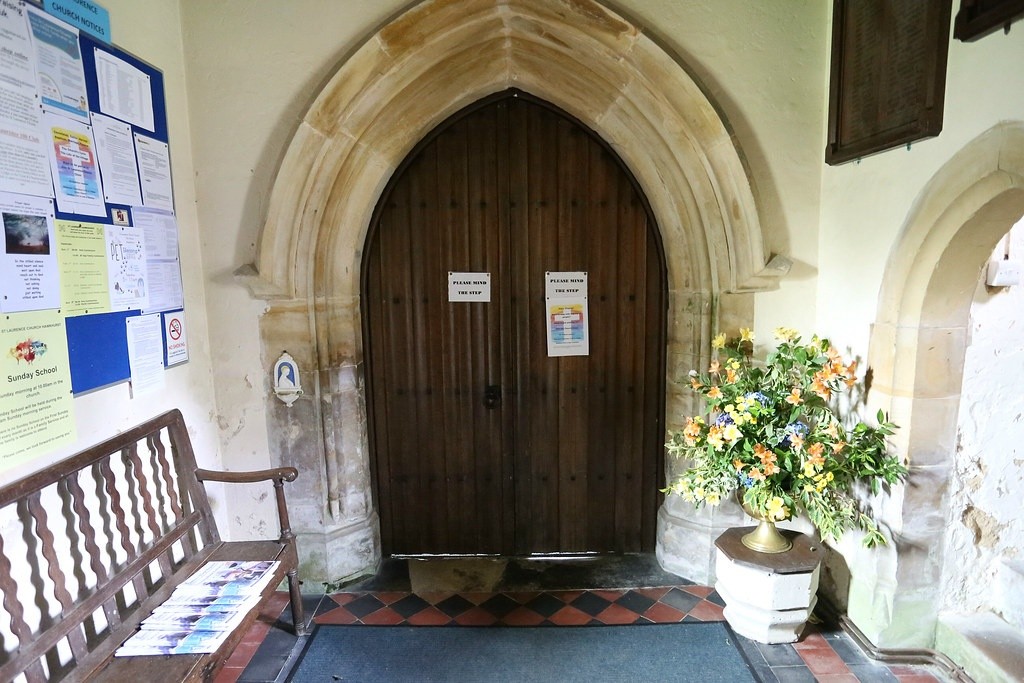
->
[114,557,281,657]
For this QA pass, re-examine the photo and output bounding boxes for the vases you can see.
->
[735,480,799,553]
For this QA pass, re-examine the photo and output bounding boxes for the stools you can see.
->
[714,527,822,644]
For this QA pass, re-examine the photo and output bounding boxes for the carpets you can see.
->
[284,621,762,683]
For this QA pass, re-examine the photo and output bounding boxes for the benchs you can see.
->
[0,408,306,683]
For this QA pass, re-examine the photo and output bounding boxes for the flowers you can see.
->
[661,326,912,549]
